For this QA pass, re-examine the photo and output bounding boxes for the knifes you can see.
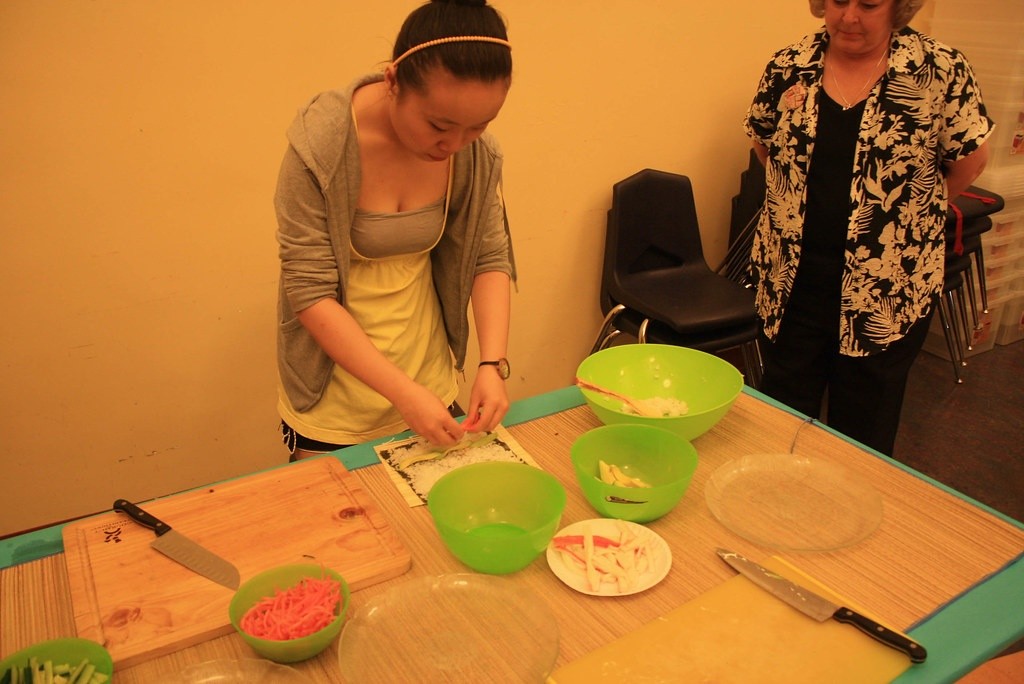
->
[113,499,240,591]
[714,548,927,664]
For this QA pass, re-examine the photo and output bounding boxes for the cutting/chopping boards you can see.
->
[62,457,411,672]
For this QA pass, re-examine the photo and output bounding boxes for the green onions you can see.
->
[603,358,690,417]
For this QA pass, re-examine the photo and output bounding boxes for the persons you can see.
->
[272,0,520,461]
[745,0,997,456]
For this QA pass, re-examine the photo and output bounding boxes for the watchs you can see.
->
[479,358,510,379]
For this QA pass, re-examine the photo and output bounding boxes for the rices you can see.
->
[386,429,527,500]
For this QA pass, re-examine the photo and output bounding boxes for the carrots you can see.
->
[239,560,343,641]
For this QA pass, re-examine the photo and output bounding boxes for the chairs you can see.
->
[590,144,1004,388]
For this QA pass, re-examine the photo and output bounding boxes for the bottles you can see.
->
[1008,112,1024,154]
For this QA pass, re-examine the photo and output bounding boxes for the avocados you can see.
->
[599,460,652,487]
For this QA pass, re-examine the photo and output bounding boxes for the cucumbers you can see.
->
[0,656,109,684]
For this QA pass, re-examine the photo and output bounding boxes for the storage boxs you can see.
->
[921,163,1024,363]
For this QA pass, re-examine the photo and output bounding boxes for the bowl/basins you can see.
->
[428,461,566,573]
[571,423,699,523]
[229,564,350,662]
[0,637,113,684]
[576,343,744,441]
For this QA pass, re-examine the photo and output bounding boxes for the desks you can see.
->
[1,377,1024,684]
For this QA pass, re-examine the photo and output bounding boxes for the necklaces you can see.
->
[828,49,887,110]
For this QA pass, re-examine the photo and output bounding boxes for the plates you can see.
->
[704,454,883,553]
[172,659,314,684]
[546,518,672,596]
[337,573,559,683]
